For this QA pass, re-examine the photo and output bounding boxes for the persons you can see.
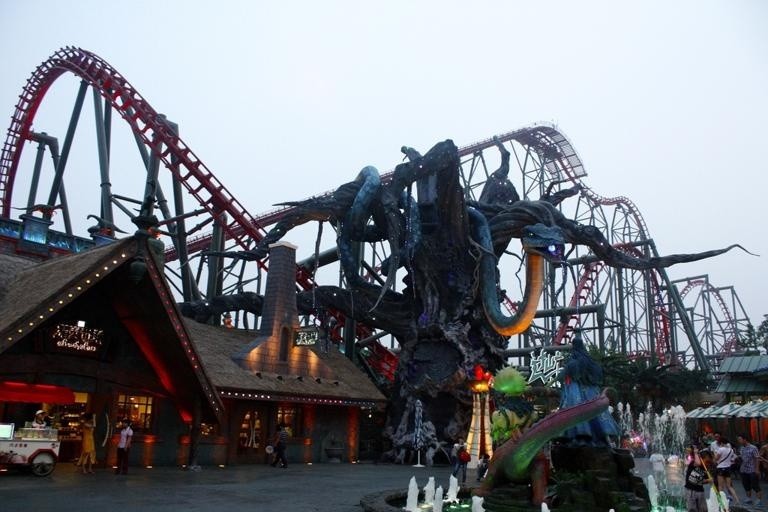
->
[60,415,70,427]
[621,435,634,457]
[476,454,490,480]
[32,410,54,428]
[271,424,288,468]
[77,413,96,475]
[453,436,467,486]
[113,420,133,475]
[650,431,768,512]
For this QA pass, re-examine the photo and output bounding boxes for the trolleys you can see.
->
[0,422,61,478]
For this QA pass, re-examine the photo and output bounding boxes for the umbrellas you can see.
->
[413,400,424,464]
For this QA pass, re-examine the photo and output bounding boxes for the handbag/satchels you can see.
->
[459,450,471,462]
